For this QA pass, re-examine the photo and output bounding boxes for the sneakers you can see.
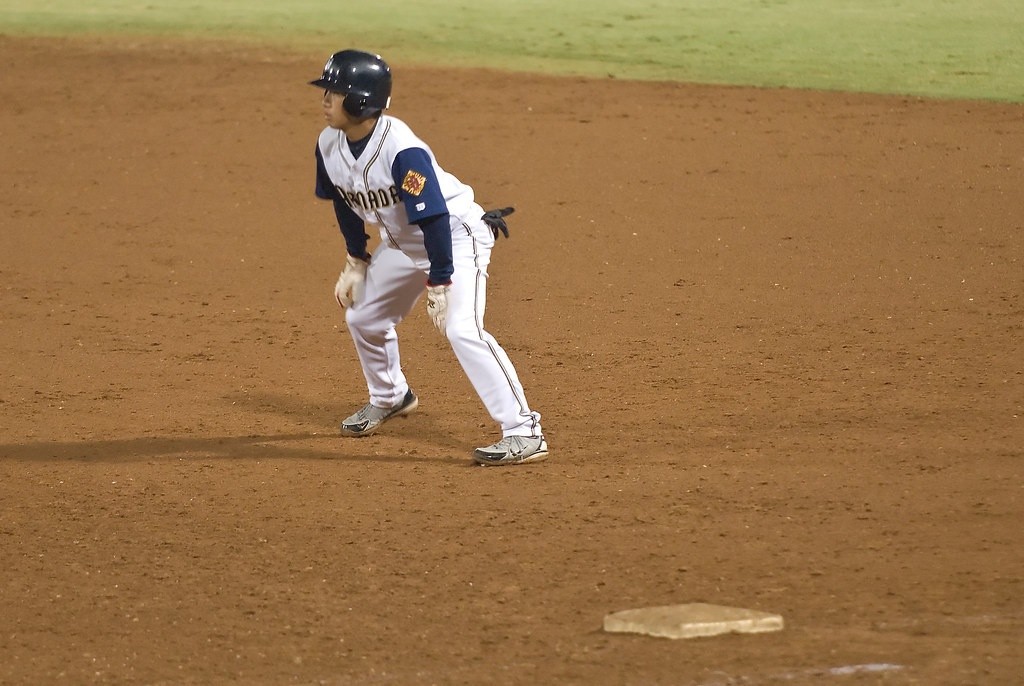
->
[472,435,549,466]
[340,388,418,437]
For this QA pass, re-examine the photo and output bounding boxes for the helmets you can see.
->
[310,50,392,118]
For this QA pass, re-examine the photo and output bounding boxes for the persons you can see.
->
[307,49,549,467]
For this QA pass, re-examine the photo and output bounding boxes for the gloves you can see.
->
[481,207,515,241]
[425,278,452,336]
[334,251,372,309]
